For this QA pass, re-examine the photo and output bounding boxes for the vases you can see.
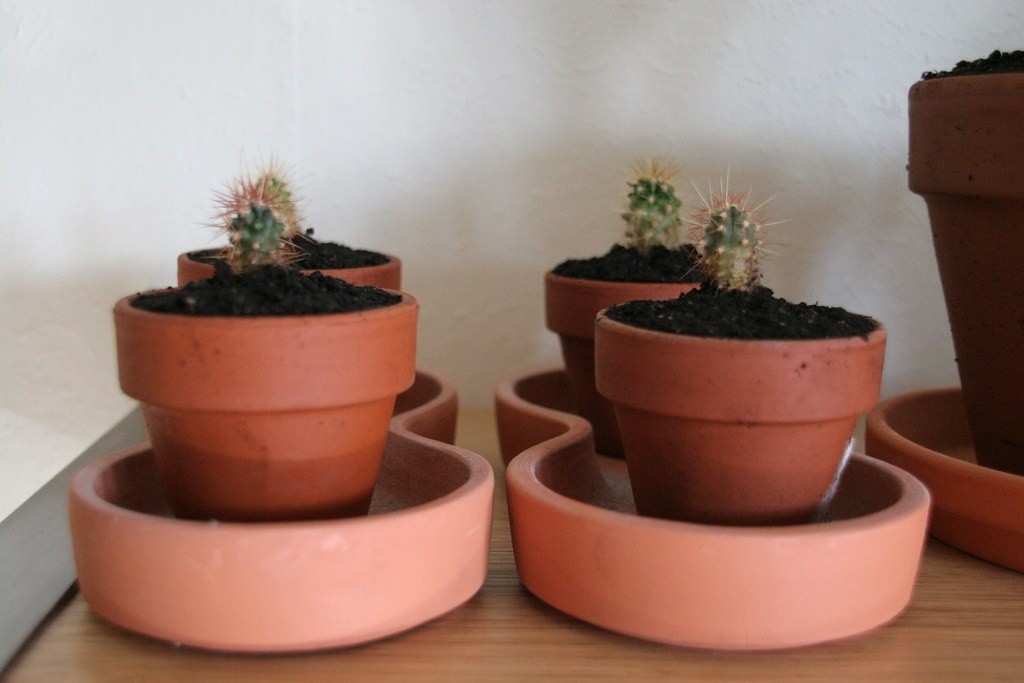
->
[908,75,1024,474]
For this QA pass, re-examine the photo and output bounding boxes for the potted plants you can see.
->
[542,147,705,463]
[111,157,421,526]
[593,163,887,526]
